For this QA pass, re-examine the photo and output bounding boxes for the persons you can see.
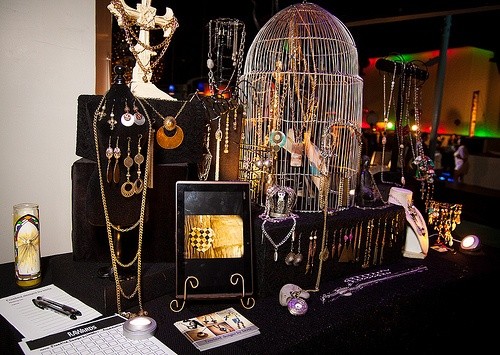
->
[386,186,428,259]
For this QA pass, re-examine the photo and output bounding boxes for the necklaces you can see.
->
[383,63,433,235]
[260,218,297,262]
[93,91,189,317]
[110,0,177,83]
[206,19,248,103]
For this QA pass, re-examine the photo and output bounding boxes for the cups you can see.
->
[13,202,41,290]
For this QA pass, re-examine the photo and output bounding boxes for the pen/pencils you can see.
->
[32,296,82,320]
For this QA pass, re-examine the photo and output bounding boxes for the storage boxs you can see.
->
[72,160,201,263]
[76,95,198,167]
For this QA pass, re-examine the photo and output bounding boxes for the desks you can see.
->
[0,247,470,355]
